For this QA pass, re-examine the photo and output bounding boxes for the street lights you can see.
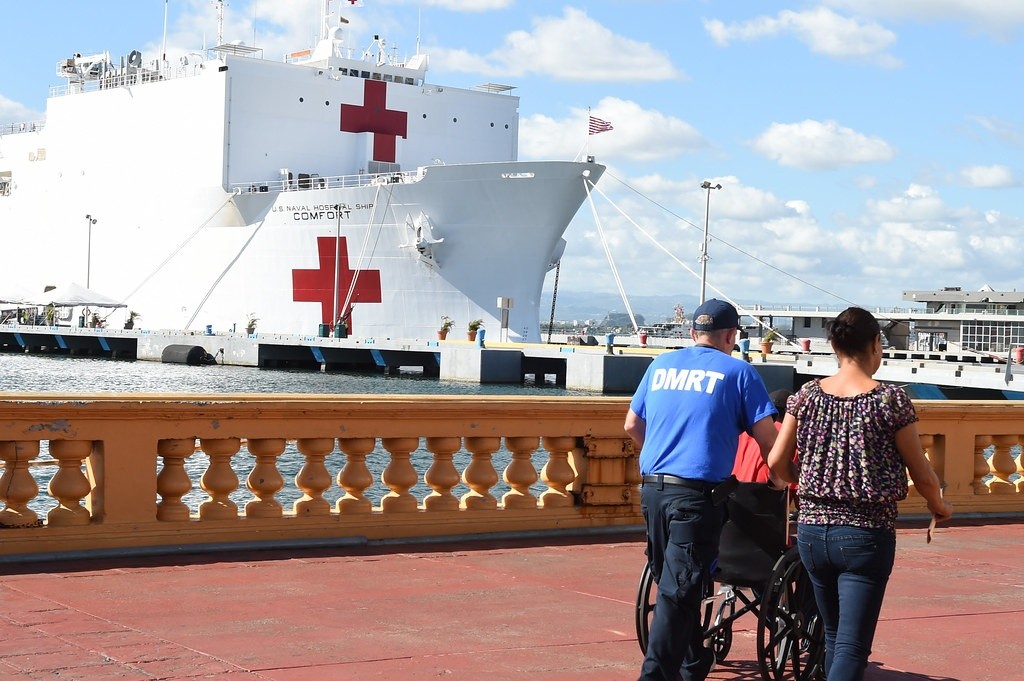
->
[84,214,97,328]
[700,181,722,306]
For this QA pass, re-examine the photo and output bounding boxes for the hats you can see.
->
[769,389,793,409]
[693,298,745,333]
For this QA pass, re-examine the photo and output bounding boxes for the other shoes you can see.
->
[705,647,716,672]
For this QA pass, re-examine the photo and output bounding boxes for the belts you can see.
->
[644,475,715,490]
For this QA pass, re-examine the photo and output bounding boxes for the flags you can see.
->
[589,116,613,135]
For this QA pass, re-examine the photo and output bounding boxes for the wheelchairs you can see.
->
[635,479,826,681]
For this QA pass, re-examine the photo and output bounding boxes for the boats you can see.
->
[0,1,607,345]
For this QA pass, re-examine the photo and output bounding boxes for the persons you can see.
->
[624,299,788,681]
[767,307,953,681]
[735,389,801,546]
